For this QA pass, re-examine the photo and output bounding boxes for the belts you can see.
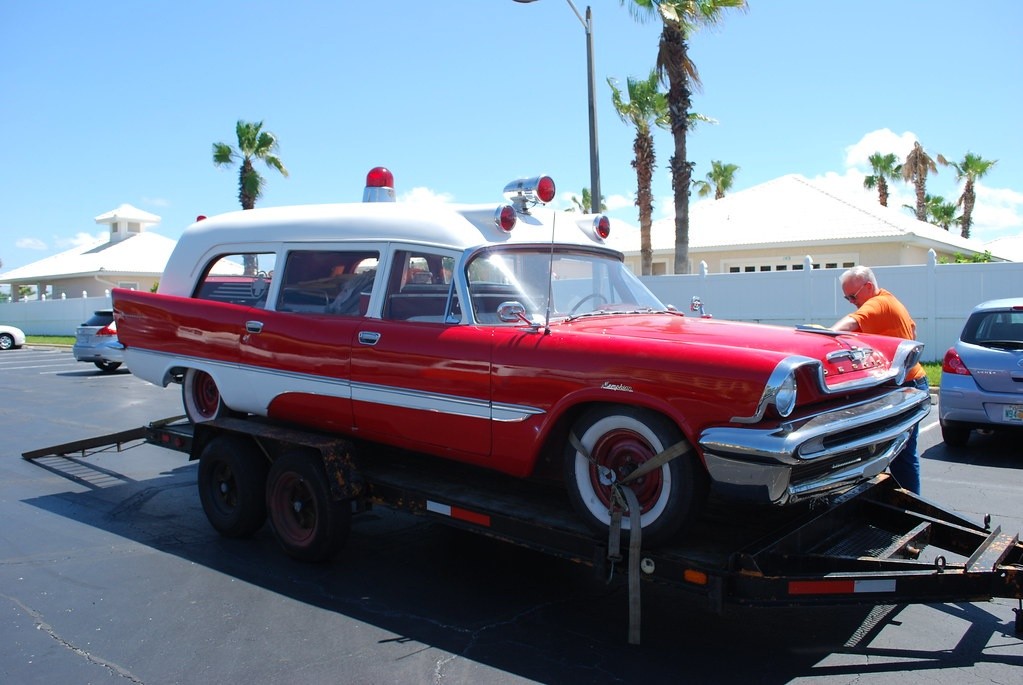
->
[906,376,926,386]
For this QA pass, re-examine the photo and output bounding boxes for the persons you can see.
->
[829,265,929,499]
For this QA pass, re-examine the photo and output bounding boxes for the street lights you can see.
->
[512,0,607,313]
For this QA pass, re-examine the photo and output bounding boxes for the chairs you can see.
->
[990,323,1023,341]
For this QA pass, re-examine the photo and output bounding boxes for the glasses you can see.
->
[843,279,869,300]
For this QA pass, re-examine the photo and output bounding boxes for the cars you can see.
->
[937,296,1023,447]
[0,325,26,350]
[108,167,933,557]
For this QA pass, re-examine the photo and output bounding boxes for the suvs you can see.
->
[72,309,124,372]
